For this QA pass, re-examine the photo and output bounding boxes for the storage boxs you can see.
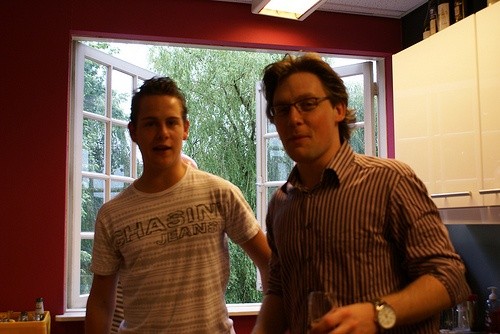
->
[0,311,52,334]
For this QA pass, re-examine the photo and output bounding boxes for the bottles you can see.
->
[425,23,429,32]
[35,297,44,320]
[1,309,44,321]
[454,0,464,23]
[437,0,450,33]
[429,8,437,35]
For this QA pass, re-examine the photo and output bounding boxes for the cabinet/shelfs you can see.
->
[391,2,500,224]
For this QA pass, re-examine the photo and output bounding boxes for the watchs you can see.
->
[368,298,397,333]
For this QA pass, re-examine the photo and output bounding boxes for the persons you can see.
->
[249,50,472,334]
[84,75,272,334]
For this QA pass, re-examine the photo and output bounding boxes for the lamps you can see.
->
[251,0,327,22]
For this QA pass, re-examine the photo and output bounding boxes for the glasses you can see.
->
[268,97,327,118]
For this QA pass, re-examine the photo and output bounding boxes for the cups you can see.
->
[308,291,339,334]
